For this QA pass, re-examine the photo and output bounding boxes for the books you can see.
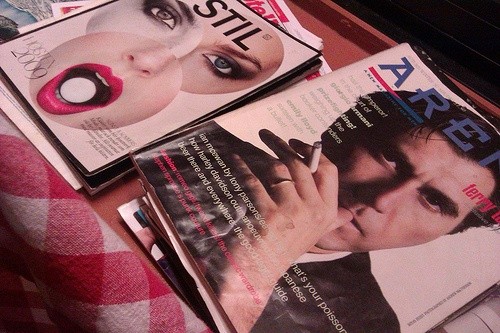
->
[0,0,500,333]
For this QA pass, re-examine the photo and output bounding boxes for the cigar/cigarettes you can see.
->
[308,141,324,174]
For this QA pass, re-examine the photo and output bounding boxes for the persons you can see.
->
[29,0,285,132]
[132,89,500,333]
